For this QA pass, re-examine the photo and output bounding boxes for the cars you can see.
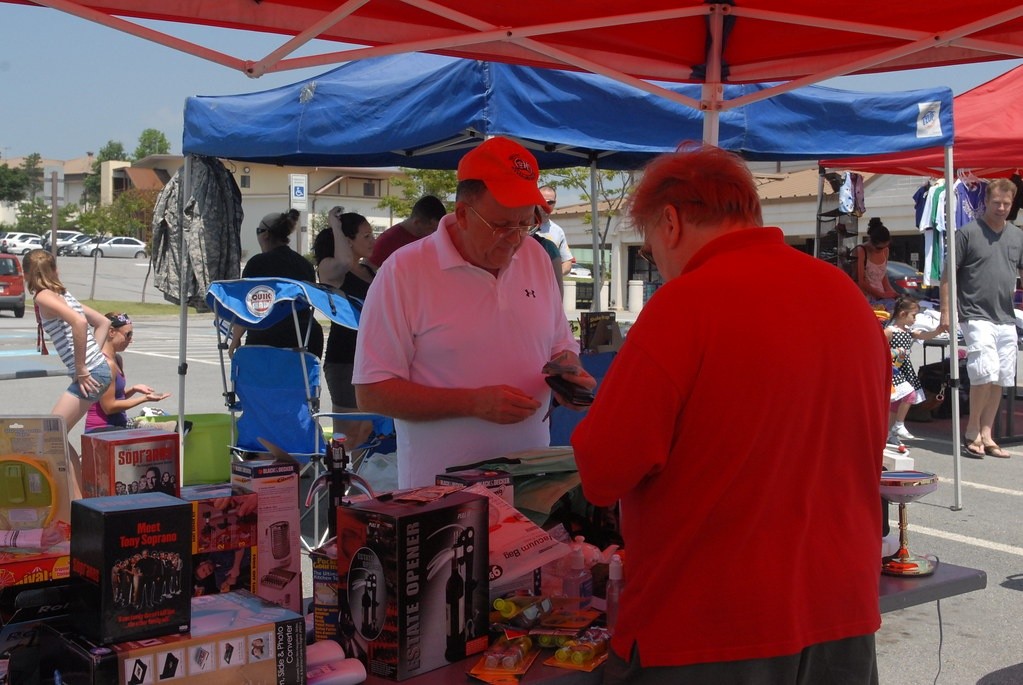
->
[79,236,148,259]
[7,237,44,255]
[884,261,932,298]
[48,234,85,256]
[63,235,113,257]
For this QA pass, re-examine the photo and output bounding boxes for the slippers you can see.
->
[982,443,1009,459]
[961,432,985,458]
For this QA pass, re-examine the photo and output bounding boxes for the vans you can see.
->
[0,253,25,317]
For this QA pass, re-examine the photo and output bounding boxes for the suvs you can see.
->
[41,230,83,250]
[0,231,41,252]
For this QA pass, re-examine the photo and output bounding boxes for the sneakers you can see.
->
[891,423,914,439]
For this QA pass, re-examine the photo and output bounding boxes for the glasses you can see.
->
[465,202,540,244]
[114,327,133,341]
[544,199,555,206]
[638,213,668,266]
[257,227,267,234]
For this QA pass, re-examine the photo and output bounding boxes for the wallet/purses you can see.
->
[545,375,594,406]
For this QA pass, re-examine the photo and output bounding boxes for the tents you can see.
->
[0,0,1023,511]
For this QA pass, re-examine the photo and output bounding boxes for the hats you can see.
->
[458,137,552,218]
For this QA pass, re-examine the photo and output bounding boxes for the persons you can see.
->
[360,195,447,271]
[530,183,573,302]
[938,177,1023,457]
[0,240,8,254]
[227,209,323,356]
[855,218,898,303]
[570,143,891,685]
[83,311,171,435]
[887,558,918,572]
[22,249,111,499]
[884,296,944,445]
[112,549,182,609]
[115,468,178,498]
[350,136,595,493]
[310,202,378,473]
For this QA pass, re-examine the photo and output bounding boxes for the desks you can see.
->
[913,323,1023,445]
[359,539,987,685]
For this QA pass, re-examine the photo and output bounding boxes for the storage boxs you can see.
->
[0,414,619,685]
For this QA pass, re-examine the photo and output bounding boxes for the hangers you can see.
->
[929,167,980,188]
[841,170,865,179]
[192,154,236,173]
